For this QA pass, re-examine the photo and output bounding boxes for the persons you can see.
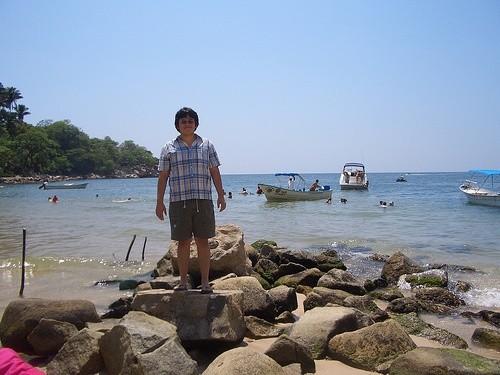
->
[343,169,364,184]
[309,180,322,191]
[326,197,394,208]
[38,182,59,202]
[287,175,296,191]
[155,107,227,294]
[222,185,262,199]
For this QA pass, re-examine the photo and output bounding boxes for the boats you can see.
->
[459,169,500,207]
[258,174,332,202]
[339,163,369,190]
[37,181,89,189]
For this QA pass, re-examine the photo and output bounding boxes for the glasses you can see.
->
[178,119,196,124]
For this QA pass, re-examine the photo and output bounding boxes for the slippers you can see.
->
[201,283,213,294]
[174,284,188,290]
